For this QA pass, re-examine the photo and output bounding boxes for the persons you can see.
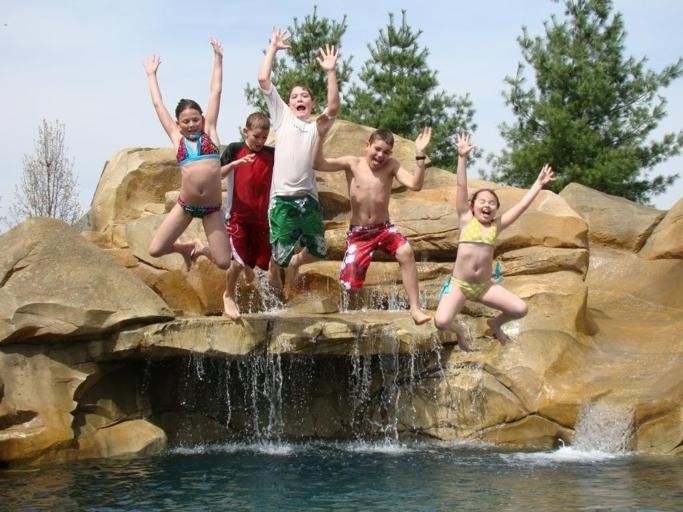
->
[312,126,431,325]
[144,35,232,269]
[258,26,338,299]
[434,130,557,352]
[218,112,275,320]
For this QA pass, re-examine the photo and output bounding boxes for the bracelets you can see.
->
[414,155,426,160]
